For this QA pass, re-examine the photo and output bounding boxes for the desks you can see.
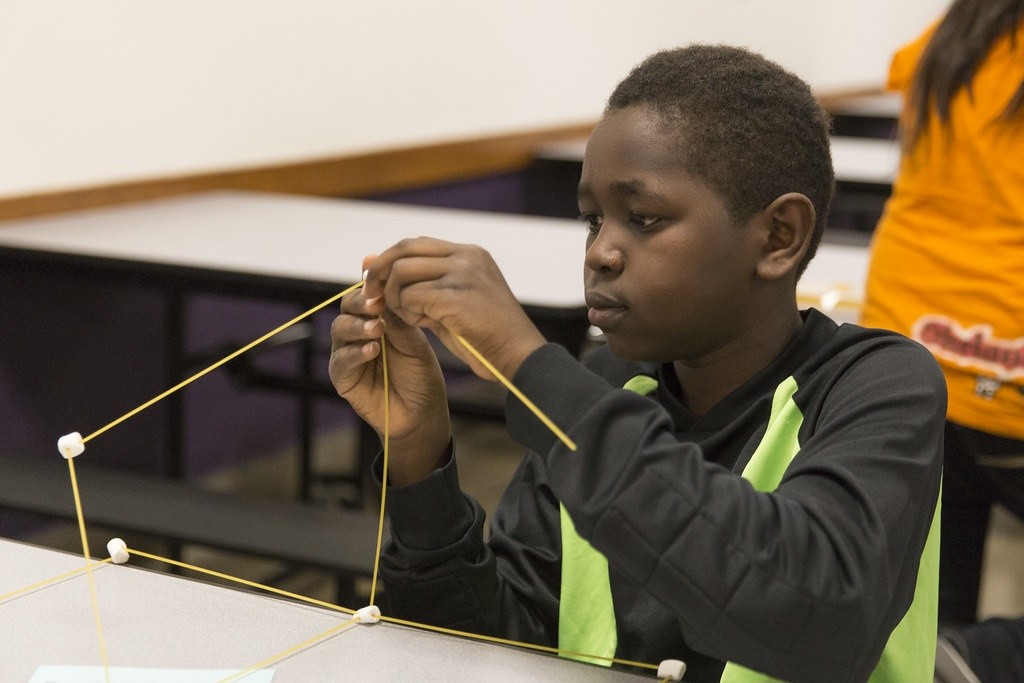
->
[534,132,899,214]
[0,190,874,575]
[0,538,673,683]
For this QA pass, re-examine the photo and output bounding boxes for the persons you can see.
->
[860,0,1023,682]
[327,47,948,683]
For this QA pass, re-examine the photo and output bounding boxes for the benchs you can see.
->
[0,458,392,577]
